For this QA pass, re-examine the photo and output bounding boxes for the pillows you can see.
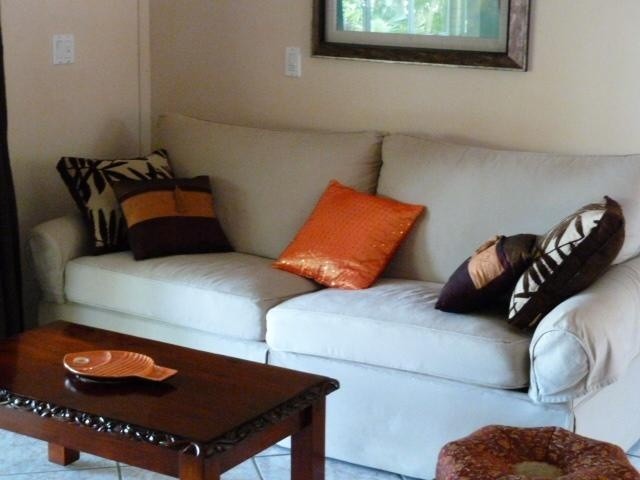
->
[53,146,234,263]
[434,195,627,333]
[270,178,426,290]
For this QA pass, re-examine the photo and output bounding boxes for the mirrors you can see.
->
[309,1,533,74]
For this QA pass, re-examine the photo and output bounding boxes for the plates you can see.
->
[63,351,177,383]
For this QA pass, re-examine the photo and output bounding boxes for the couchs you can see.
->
[12,110,640,479]
